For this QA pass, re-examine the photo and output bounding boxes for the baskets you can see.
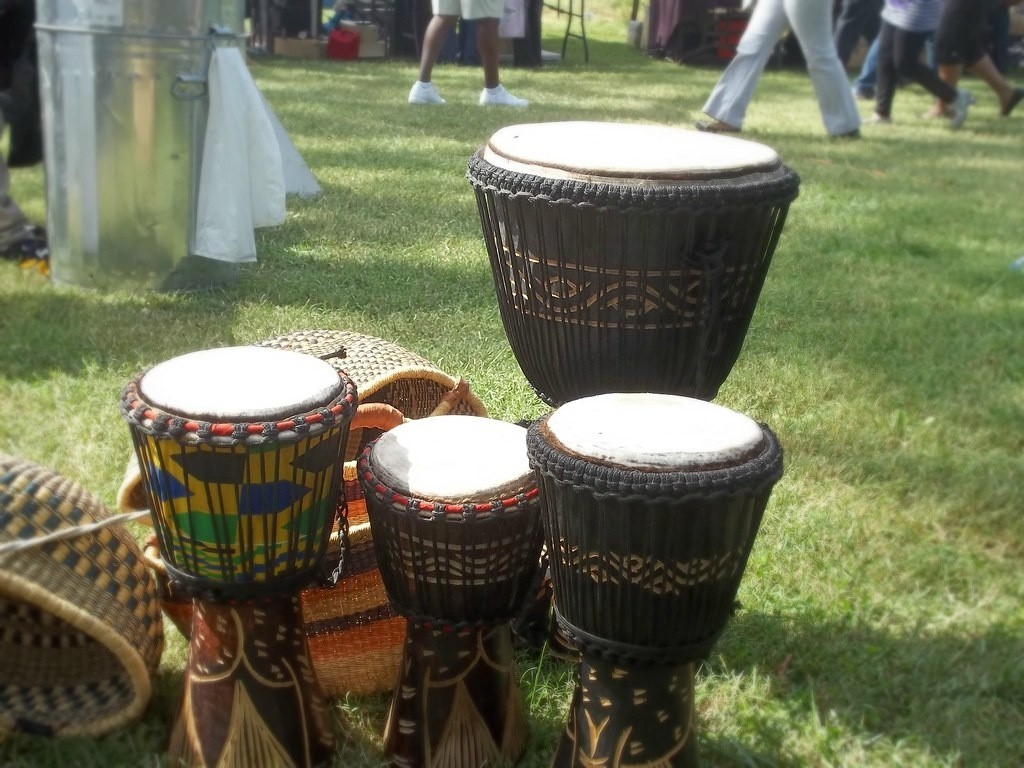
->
[116,328,488,699]
[0,453,165,768]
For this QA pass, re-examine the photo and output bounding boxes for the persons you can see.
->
[0,0,48,262]
[834,0,1024,119]
[861,0,972,131]
[695,0,862,141]
[407,0,528,108]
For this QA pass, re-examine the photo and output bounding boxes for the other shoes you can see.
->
[829,128,861,142]
[862,112,891,125]
[920,108,953,120]
[999,81,1024,115]
[947,89,974,131]
[696,118,741,131]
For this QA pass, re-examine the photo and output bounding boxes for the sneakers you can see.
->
[408,82,445,105]
[479,88,527,107]
